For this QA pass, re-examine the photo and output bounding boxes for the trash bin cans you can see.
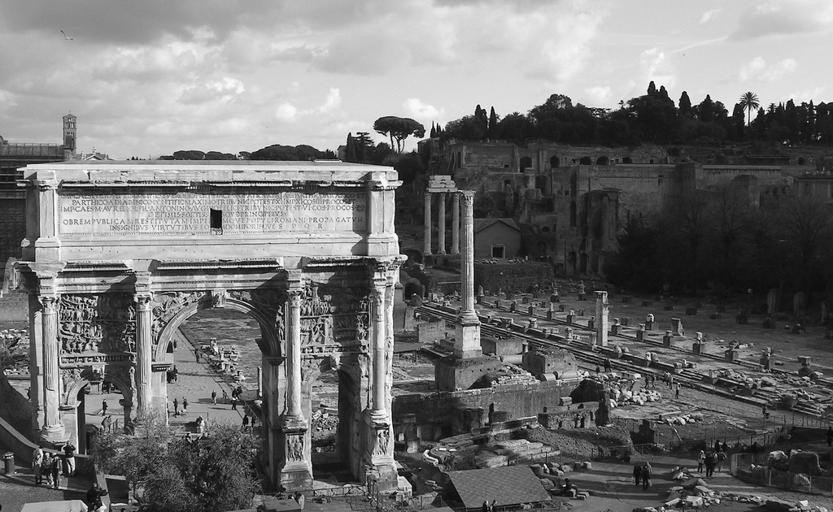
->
[0,452,14,474]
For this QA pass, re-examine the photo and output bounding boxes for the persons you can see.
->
[664,369,682,400]
[102,399,107,415]
[172,398,179,415]
[63,440,76,474]
[697,439,728,479]
[87,481,107,512]
[482,499,489,512]
[182,395,188,409]
[604,358,612,372]
[212,390,217,405]
[565,478,572,490]
[633,460,654,491]
[243,415,248,426]
[489,500,497,512]
[32,448,61,490]
[185,432,191,443]
[232,386,242,410]
